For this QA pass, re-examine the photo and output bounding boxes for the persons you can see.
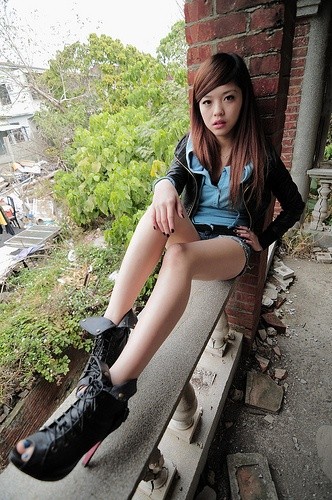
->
[11,53,306,483]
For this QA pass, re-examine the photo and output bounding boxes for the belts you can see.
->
[193,223,242,236]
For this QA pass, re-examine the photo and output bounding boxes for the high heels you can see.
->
[74,308,139,383]
[9,379,138,482]
